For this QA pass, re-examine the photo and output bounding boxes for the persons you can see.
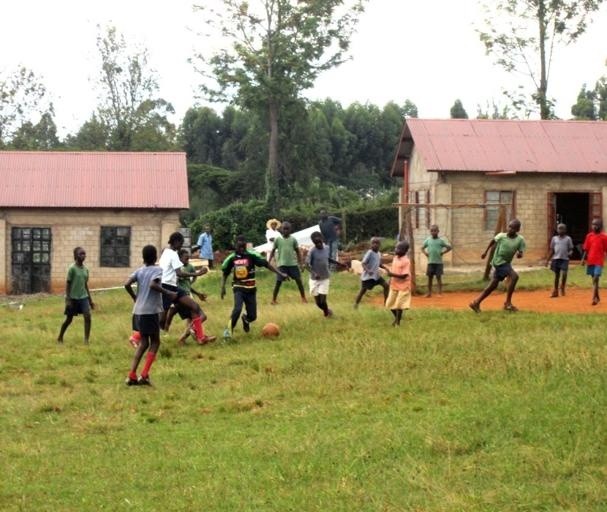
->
[129,207,348,348]
[124,244,179,385]
[57,247,96,344]
[469,219,527,312]
[421,224,453,297]
[580,217,606,305]
[385,240,412,327]
[353,235,390,308]
[545,222,575,299]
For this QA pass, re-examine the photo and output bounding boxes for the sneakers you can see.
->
[129,337,139,348]
[125,377,138,386]
[197,336,216,346]
[242,315,249,332]
[469,301,481,314]
[504,303,517,312]
[138,376,151,386]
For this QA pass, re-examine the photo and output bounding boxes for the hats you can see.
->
[266,219,282,229]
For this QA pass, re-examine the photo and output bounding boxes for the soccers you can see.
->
[262,323,280,338]
